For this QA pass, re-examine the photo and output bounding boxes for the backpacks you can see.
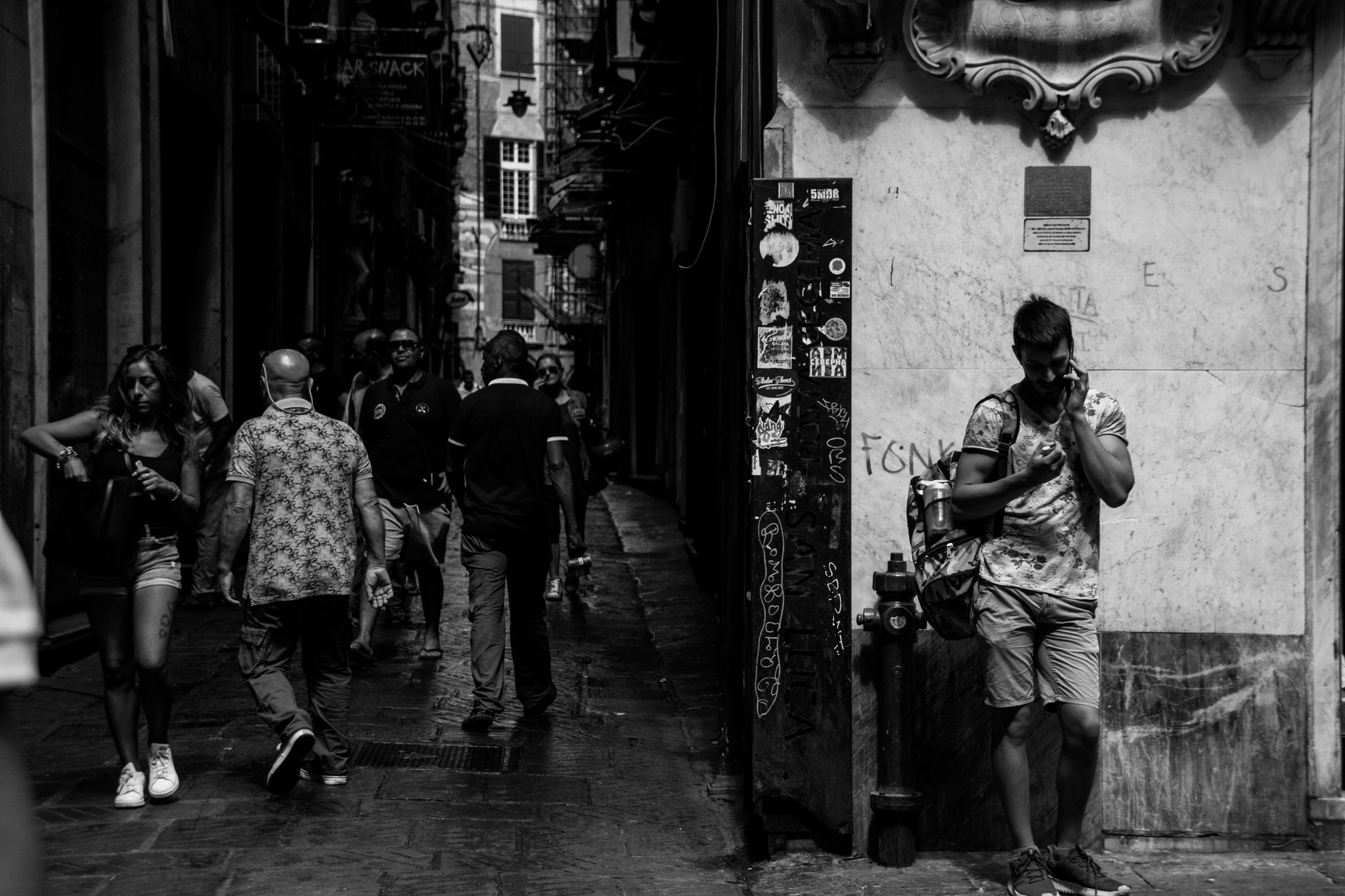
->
[906,387,1022,646]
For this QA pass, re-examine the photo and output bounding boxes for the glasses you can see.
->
[388,340,419,353]
[537,366,560,375]
[127,344,168,358]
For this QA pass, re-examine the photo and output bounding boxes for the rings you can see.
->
[146,473,150,479]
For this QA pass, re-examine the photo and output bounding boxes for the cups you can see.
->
[923,483,952,537]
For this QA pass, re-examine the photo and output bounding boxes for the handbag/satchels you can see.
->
[38,400,142,585]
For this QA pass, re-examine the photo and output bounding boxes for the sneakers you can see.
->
[1047,843,1130,896]
[113,761,145,807]
[564,559,580,592]
[145,741,180,799]
[546,578,563,600]
[1007,848,1060,896]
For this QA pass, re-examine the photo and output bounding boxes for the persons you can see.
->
[350,326,461,660]
[0,507,42,896]
[533,353,601,600]
[296,331,348,420]
[158,346,235,610]
[443,329,584,731]
[19,345,202,809]
[341,327,394,626]
[953,295,1136,896]
[456,369,483,400]
[394,535,420,595]
[214,347,395,795]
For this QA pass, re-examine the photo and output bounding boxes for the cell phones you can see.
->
[1066,342,1074,397]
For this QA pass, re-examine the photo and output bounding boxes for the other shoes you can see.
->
[264,727,316,795]
[407,583,421,597]
[461,708,496,733]
[385,604,410,625]
[190,591,228,608]
[321,769,349,785]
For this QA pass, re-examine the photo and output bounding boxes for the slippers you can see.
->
[350,641,376,661]
[418,646,443,661]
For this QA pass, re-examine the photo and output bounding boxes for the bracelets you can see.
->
[168,482,180,502]
[57,446,80,474]
